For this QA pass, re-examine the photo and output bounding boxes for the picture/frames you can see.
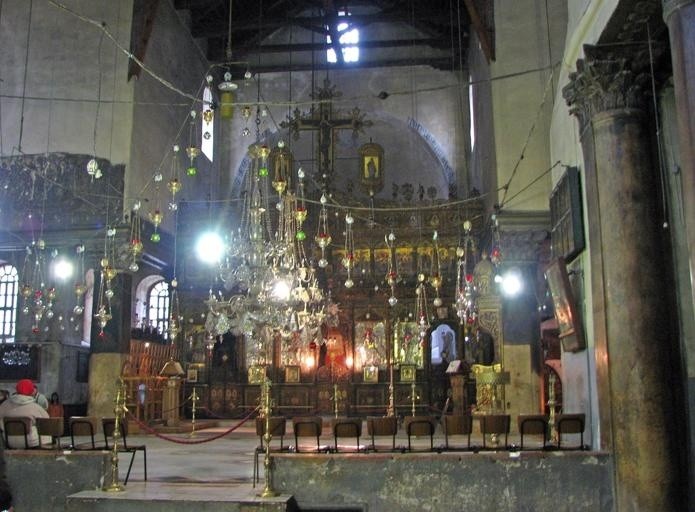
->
[185,367,200,384]
[361,365,379,383]
[75,350,88,383]
[247,364,270,384]
[398,364,417,382]
[284,366,302,384]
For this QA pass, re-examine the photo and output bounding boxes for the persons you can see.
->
[32,385,49,411]
[0,379,53,449]
[48,392,64,417]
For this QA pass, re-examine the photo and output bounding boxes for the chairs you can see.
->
[512,414,553,451]
[479,415,512,451]
[328,418,368,454]
[252,416,292,487]
[440,415,476,452]
[291,416,330,454]
[3,415,147,485]
[552,414,586,452]
[403,416,440,452]
[365,416,405,453]
